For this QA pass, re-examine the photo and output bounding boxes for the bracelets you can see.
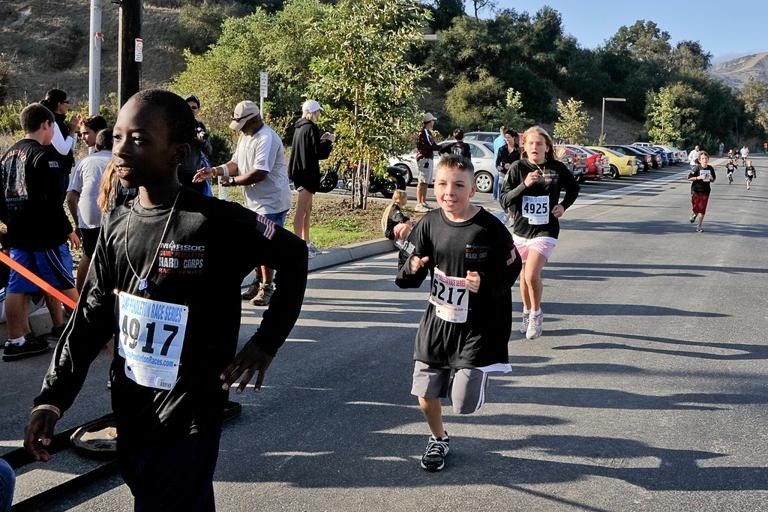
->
[695,176,698,181]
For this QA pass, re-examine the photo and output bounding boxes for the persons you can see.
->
[0,88,136,363]
[23,86,311,511]
[186,95,337,306]
[493,125,581,341]
[393,154,523,472]
[688,150,716,232]
[718,141,768,190]
[381,111,470,250]
[688,145,701,171]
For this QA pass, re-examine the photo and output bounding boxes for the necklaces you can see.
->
[125,184,181,289]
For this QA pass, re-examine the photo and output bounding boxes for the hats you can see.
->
[229,100,259,131]
[301,99,325,113]
[422,112,438,125]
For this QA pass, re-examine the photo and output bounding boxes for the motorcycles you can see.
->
[313,148,410,201]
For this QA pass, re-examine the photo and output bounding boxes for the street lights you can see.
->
[350,25,443,141]
[598,95,626,145]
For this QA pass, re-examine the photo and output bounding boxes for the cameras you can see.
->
[196,128,209,141]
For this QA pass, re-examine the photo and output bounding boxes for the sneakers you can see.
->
[304,240,321,258]
[415,202,435,214]
[519,308,532,335]
[2,337,54,362]
[525,309,545,343]
[420,432,452,474]
[241,280,275,307]
